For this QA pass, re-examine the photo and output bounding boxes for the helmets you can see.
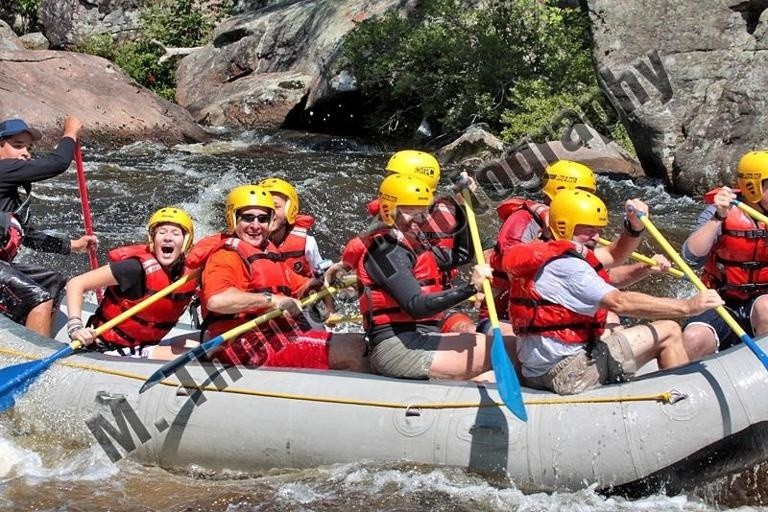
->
[385,150,440,189]
[736,151,768,203]
[543,160,597,200]
[543,189,608,240]
[379,174,440,226]
[147,177,299,253]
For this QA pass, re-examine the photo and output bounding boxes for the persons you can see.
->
[501,188,726,395]
[258,178,336,331]
[673,150,768,363]
[0,114,98,338]
[478,159,671,336]
[326,150,477,333]
[342,173,523,383]
[183,185,374,373]
[65,207,204,358]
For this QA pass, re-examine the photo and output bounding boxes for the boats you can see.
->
[1,296,767,498]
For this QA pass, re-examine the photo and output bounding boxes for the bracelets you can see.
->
[714,210,728,223]
[68,316,83,336]
[622,218,646,237]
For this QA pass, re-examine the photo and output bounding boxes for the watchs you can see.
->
[262,292,272,304]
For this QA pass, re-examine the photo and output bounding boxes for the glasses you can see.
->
[241,214,269,222]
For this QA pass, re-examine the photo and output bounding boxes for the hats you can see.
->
[0,119,42,140]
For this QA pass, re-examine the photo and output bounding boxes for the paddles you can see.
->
[141,274,359,394]
[0,266,204,411]
[461,171,528,422]
